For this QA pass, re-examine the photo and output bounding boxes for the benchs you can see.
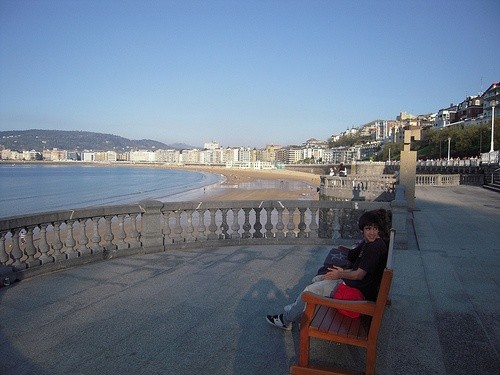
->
[288,228,396,375]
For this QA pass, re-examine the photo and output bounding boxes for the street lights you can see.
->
[489,100,497,152]
[478,113,485,154]
[447,136,452,160]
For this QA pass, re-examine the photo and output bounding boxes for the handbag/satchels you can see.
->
[334,283,365,318]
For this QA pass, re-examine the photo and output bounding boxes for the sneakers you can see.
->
[265,314,293,331]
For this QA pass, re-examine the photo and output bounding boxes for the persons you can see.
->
[330,163,348,177]
[266,209,390,330]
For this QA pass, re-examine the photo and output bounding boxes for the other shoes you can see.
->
[284,303,306,312]
